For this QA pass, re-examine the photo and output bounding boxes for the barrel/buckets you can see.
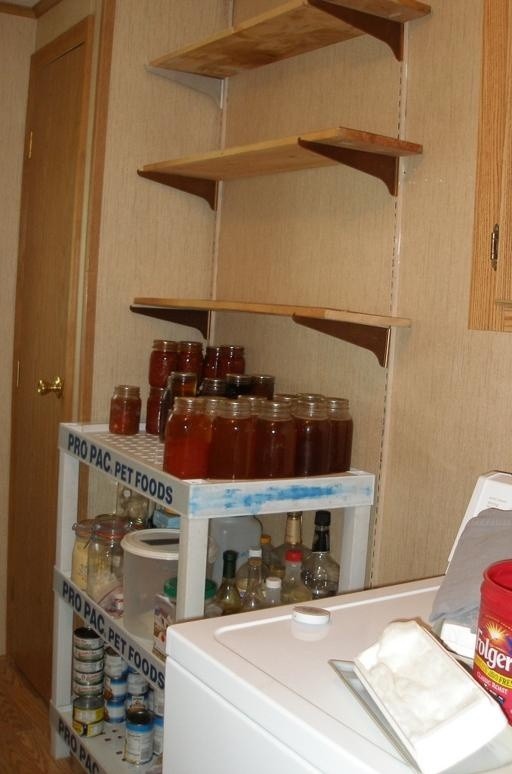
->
[119,527,207,640]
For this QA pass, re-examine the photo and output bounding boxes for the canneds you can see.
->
[73,627,164,765]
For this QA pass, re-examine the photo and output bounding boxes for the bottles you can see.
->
[258,534,275,567]
[213,550,242,614]
[302,510,340,598]
[235,547,272,599]
[146,337,354,478]
[108,383,141,435]
[283,550,312,603]
[241,556,265,614]
[263,576,282,608]
[277,511,311,559]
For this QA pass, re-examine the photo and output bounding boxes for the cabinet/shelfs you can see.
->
[50,421,376,773]
